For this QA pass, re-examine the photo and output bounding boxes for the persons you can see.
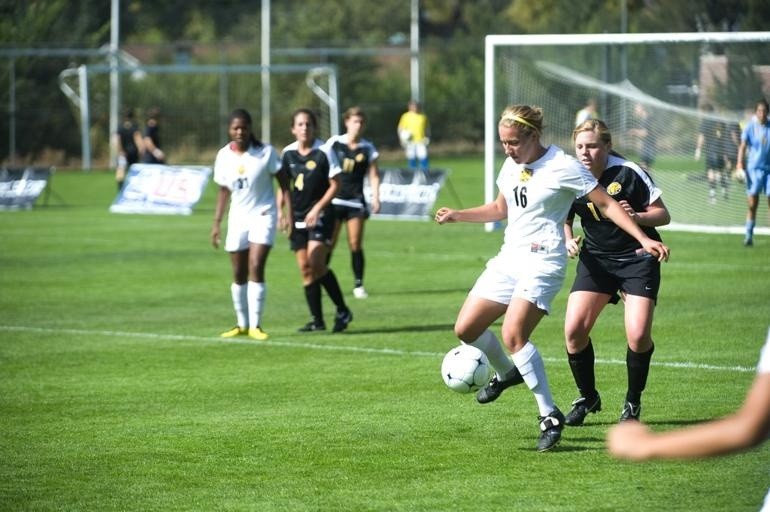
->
[397,100,429,176]
[325,108,382,299]
[606,323,770,512]
[735,100,770,247]
[434,103,672,451]
[115,108,144,190]
[692,102,729,204]
[563,116,671,426]
[574,97,600,129]
[628,103,657,166]
[722,120,747,181]
[144,109,165,164]
[280,109,354,334]
[211,108,295,341]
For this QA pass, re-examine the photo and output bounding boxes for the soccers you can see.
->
[440,344,490,394]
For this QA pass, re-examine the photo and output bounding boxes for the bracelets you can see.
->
[214,219,222,223]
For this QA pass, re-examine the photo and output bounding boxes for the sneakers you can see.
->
[331,304,354,332]
[298,317,326,334]
[618,388,641,423]
[533,404,567,453]
[562,389,602,427]
[708,186,760,247]
[246,326,274,341]
[473,363,524,406]
[220,325,248,338]
[351,284,368,300]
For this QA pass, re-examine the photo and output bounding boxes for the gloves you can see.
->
[734,167,749,184]
